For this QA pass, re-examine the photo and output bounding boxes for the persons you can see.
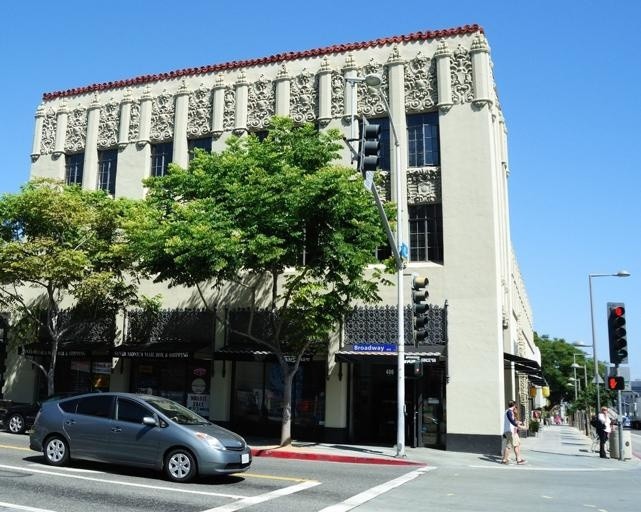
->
[545,413,551,431]
[501,401,524,465]
[596,406,612,459]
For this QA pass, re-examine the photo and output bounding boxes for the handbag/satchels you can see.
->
[590,415,606,430]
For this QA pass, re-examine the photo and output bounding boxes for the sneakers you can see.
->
[517,459,527,465]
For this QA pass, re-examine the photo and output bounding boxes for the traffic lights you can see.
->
[606,301,629,366]
[356,114,383,180]
[609,376,626,390]
[410,275,431,345]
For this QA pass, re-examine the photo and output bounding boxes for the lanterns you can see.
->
[542,386,550,397]
[530,386,537,397]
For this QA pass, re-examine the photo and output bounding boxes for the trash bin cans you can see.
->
[609,427,632,460]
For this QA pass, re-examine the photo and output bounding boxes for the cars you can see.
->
[26,392,254,484]
[3,393,93,436]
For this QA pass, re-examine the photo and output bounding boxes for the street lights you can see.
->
[588,270,633,418]
[568,377,580,398]
[572,340,594,351]
[572,351,594,405]
[567,383,575,387]
[570,362,589,411]
